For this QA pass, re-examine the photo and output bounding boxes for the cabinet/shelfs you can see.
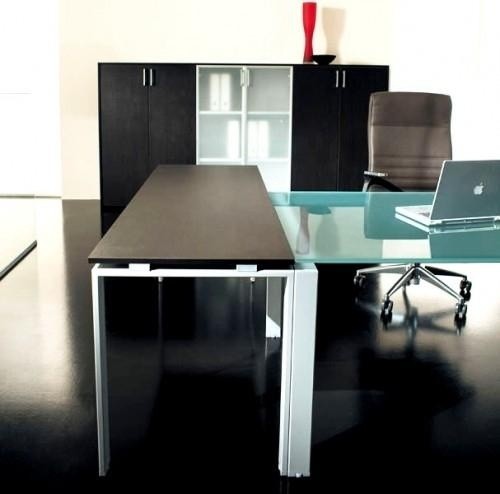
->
[100,64,195,206]
[195,64,293,191]
[291,65,389,191]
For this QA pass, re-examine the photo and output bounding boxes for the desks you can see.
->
[86,163,500,478]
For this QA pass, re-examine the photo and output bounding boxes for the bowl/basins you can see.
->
[309,54,337,66]
[308,205,330,215]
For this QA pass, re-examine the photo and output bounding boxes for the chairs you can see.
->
[354,92,471,328]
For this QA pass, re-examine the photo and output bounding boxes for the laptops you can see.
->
[395,214,500,258]
[395,160,500,226]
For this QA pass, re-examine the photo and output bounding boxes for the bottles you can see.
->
[296,205,311,255]
[301,0,318,63]
